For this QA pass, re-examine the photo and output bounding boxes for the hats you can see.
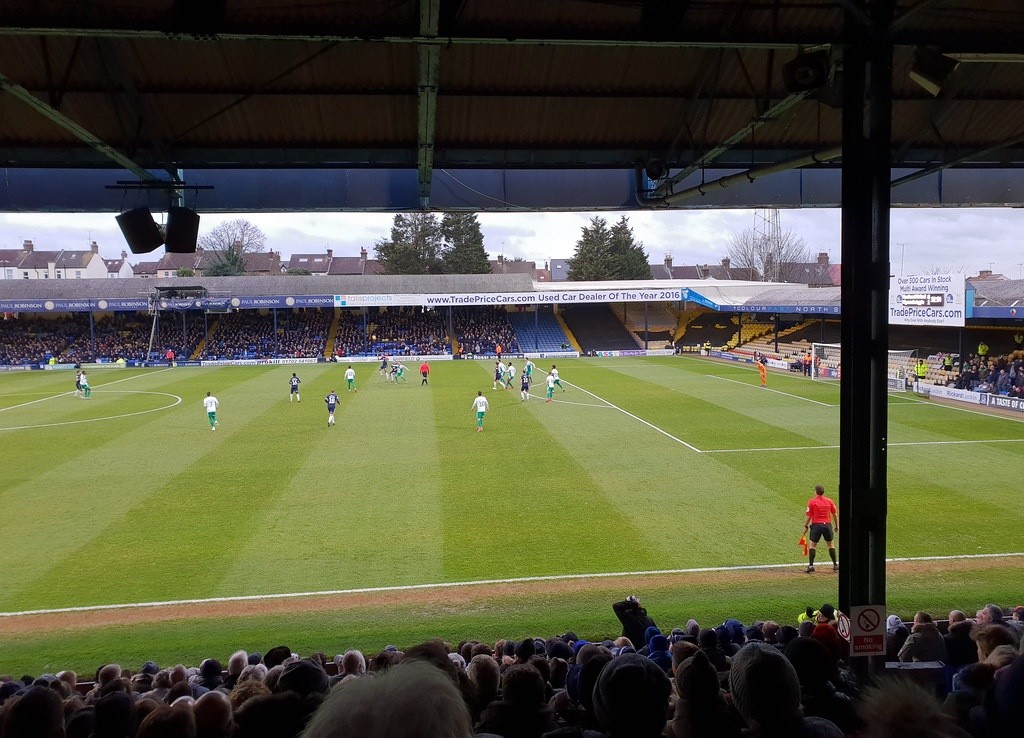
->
[0,592,1024,737]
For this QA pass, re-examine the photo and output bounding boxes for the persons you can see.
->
[420,361,430,386]
[0,305,519,367]
[204,392,219,431]
[385,361,410,385]
[803,352,820,377]
[289,373,302,403]
[74,370,92,400]
[377,354,389,380]
[705,340,711,357]
[325,390,342,427]
[470,391,489,432]
[343,366,358,393]
[915,359,927,383]
[492,357,565,403]
[942,341,1024,399]
[757,361,767,388]
[561,343,571,349]
[798,484,839,573]
[0,596,1024,738]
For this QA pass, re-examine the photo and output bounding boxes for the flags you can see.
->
[799,535,808,556]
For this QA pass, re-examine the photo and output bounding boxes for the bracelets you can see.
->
[803,525,808,528]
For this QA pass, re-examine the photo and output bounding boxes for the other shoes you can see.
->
[211,428,215,430]
[216,423,219,427]
[333,423,335,425]
[804,566,815,573]
[833,566,839,571]
[328,422,330,426]
[478,428,483,431]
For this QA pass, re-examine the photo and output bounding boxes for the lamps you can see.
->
[110,180,213,253]
[904,52,961,97]
